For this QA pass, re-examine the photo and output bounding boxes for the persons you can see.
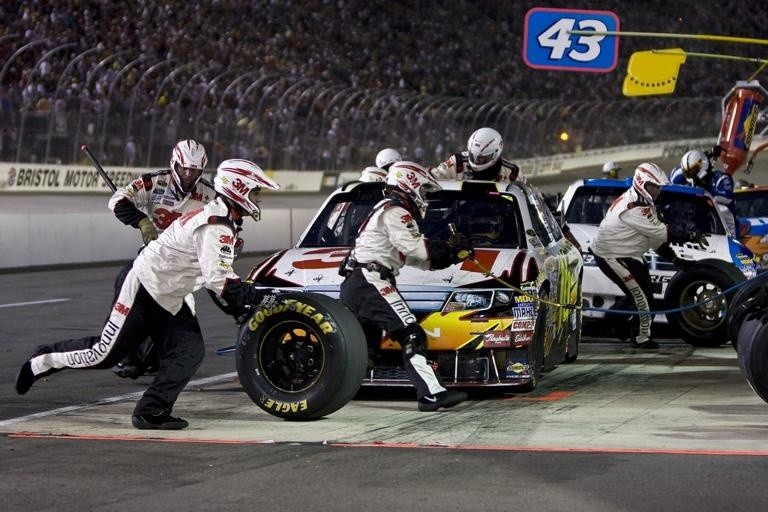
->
[588,162,696,347]
[680,150,742,245]
[110,141,201,379]
[465,211,508,244]
[318,165,385,246]
[339,162,480,410]
[428,128,513,182]
[16,160,276,430]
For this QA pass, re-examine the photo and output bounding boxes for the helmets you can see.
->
[467,126,504,174]
[360,165,387,183]
[601,160,621,175]
[632,161,671,207]
[386,160,443,221]
[169,140,208,196]
[375,148,402,168]
[679,150,712,184]
[213,157,282,222]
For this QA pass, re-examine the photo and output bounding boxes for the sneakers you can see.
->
[602,308,631,342]
[631,337,660,349]
[133,409,188,430]
[14,345,44,396]
[418,385,468,413]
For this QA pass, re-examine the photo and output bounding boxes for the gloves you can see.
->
[247,286,286,311]
[672,255,694,270]
[428,235,475,271]
[690,231,713,249]
[137,216,159,248]
[229,303,250,326]
